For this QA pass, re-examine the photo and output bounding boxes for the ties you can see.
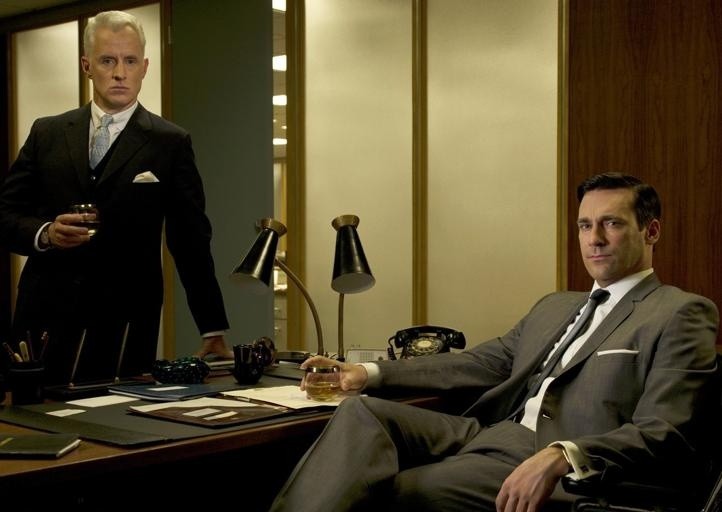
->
[487,288,611,429]
[89,114,112,170]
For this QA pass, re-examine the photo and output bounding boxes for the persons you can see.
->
[266,171,720,511]
[2,9,237,390]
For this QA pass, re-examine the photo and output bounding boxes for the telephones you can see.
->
[395,325,465,359]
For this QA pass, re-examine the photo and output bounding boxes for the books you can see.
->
[0,431,84,460]
[106,381,237,402]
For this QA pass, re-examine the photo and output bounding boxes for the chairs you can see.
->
[558,347,722,510]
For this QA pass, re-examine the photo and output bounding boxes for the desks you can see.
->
[2,383,476,509]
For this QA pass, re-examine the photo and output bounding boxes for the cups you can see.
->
[71,203,99,237]
[233,344,263,385]
[305,364,344,403]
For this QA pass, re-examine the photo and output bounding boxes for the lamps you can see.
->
[329,214,379,364]
[229,216,324,354]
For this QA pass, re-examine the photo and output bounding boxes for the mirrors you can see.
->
[0,0,428,408]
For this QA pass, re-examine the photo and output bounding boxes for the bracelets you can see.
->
[561,448,571,467]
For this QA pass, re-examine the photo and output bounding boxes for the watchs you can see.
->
[39,225,50,247]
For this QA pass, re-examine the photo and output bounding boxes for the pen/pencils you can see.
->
[3,330,50,364]
